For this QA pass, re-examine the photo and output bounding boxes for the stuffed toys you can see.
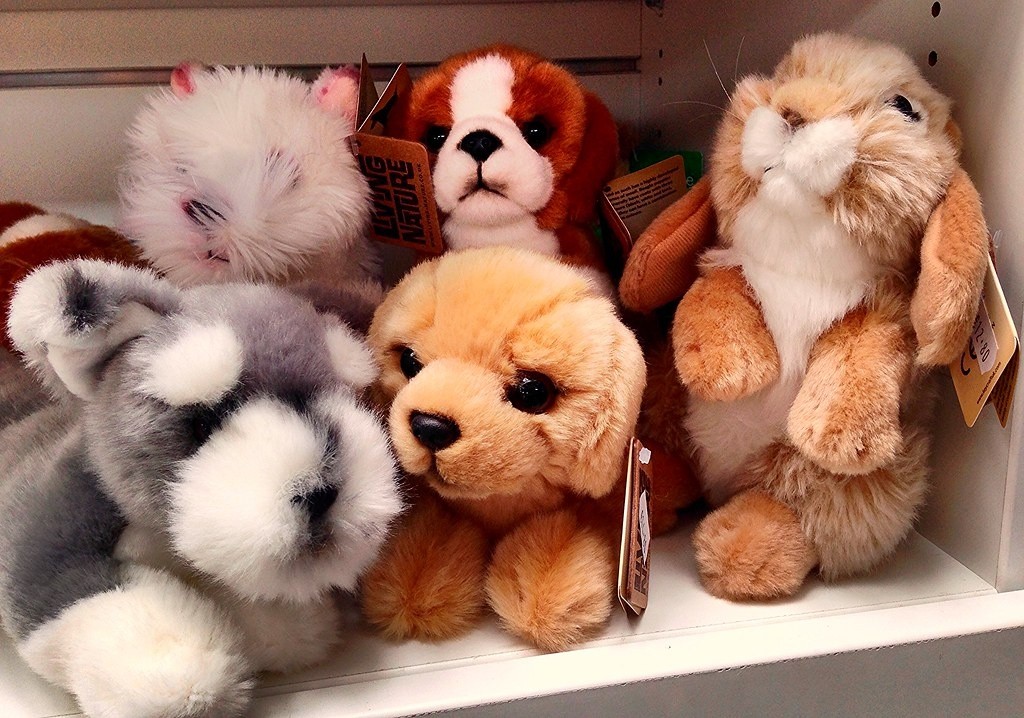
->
[0,58,370,347]
[345,247,644,653]
[623,22,991,605]
[396,35,669,351]
[0,256,411,718]
